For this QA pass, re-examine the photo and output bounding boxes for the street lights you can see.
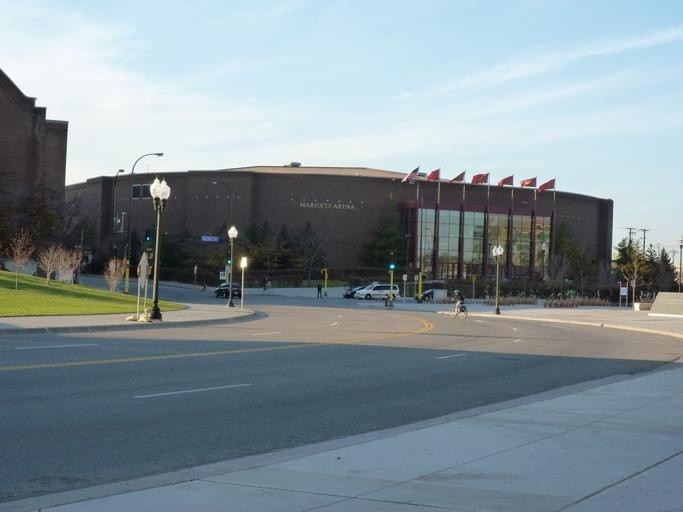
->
[388,264,396,300]
[492,244,504,307]
[112,170,126,260]
[227,225,239,300]
[240,255,248,312]
[677,234,683,284]
[150,175,171,305]
[125,153,164,294]
[211,180,232,228]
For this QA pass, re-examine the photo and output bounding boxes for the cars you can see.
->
[343,286,366,298]
[414,289,452,301]
[214,282,240,299]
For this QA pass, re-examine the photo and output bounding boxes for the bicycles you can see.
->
[448,300,468,320]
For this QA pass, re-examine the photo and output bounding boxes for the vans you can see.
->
[354,282,400,300]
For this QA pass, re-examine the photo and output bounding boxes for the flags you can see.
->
[472,174,488,184]
[426,170,439,181]
[401,167,418,183]
[497,175,513,189]
[450,171,465,182]
[538,180,556,193]
[521,177,536,189]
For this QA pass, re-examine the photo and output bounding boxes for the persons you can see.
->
[263,276,269,291]
[455,290,463,317]
[317,282,323,300]
